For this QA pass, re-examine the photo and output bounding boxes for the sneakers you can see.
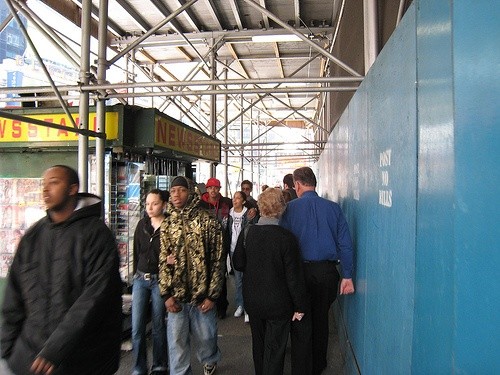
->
[203,361,216,375]
[234,305,244,317]
[244,310,249,323]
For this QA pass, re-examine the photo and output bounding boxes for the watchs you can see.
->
[254,207,257,211]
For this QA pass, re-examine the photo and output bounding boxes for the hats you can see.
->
[205,178,221,188]
[171,176,188,189]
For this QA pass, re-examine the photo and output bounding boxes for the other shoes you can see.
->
[150,370,169,375]
[131,368,148,375]
[218,311,225,319]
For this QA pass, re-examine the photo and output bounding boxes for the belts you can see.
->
[137,270,158,281]
[304,261,337,265]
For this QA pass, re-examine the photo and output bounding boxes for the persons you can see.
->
[129,166,354,375]
[0,164,122,375]
